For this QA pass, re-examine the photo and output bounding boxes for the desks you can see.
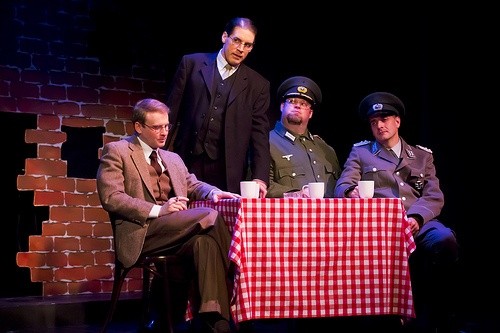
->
[185,197,416,333]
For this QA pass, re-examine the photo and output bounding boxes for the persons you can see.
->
[334,92,456,333]
[97,99,241,333]
[246,77,341,199]
[159,18,271,199]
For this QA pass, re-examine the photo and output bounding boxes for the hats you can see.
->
[359,92,405,118]
[276,76,322,111]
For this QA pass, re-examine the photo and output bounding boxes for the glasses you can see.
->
[140,121,173,132]
[285,98,311,108]
[229,35,255,49]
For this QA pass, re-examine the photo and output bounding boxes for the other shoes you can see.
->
[200,319,231,333]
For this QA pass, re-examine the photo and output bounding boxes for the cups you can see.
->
[358,180,375,198]
[302,182,325,198]
[239,181,260,198]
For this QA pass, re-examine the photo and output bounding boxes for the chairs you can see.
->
[102,212,181,333]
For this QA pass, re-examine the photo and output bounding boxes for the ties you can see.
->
[149,151,162,176]
